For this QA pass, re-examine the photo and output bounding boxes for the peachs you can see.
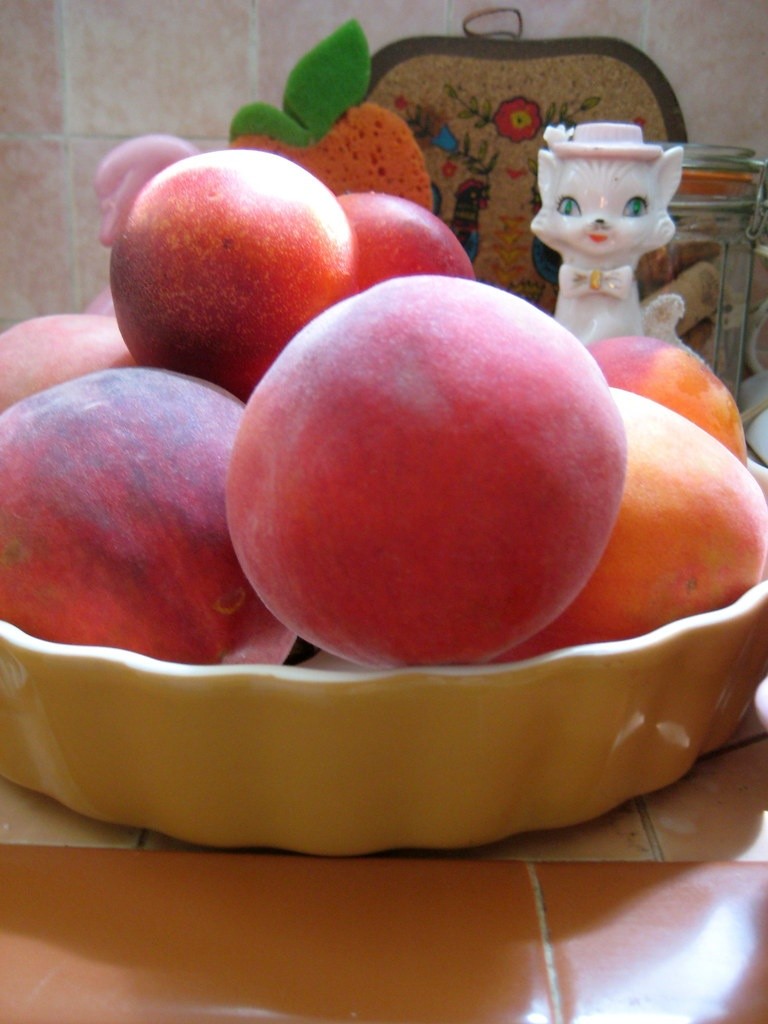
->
[0,147,767,674]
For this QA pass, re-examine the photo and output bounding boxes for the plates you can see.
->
[652,142,765,209]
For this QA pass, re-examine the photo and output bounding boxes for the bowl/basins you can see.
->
[0,460,767,854]
